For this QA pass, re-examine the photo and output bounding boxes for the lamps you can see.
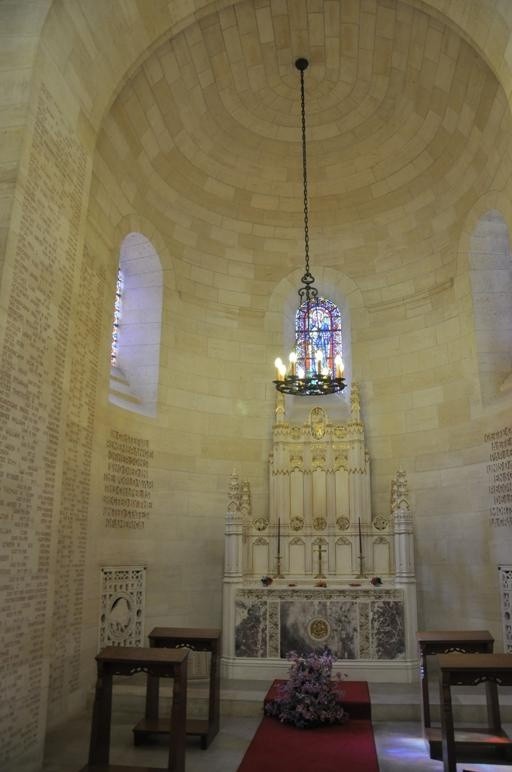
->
[272,58,347,397]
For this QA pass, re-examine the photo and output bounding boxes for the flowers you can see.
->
[264,649,351,730]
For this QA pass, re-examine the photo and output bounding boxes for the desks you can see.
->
[86,645,189,772]
[439,652,512,772]
[417,629,509,763]
[132,626,224,750]
